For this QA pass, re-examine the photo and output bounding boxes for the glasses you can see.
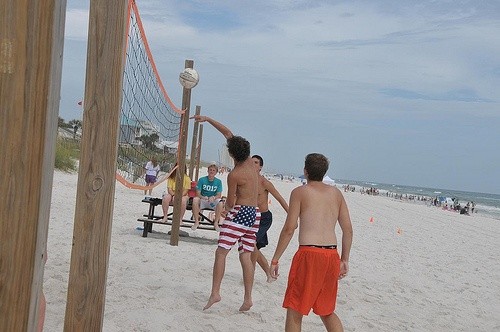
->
[255,161,261,164]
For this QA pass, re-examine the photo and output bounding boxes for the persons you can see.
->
[251,154,298,282]
[261,170,305,185]
[269,153,353,332]
[160,161,191,225]
[143,158,160,195]
[341,183,356,193]
[359,186,477,217]
[191,163,232,232]
[190,115,261,312]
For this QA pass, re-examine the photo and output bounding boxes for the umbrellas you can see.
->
[298,172,336,185]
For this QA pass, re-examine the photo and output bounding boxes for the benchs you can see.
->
[136,215,223,231]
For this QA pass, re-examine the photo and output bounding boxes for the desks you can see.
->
[142,195,213,236]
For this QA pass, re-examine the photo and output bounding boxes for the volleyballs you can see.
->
[179,68,199,89]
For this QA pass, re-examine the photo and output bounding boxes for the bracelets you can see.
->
[271,260,278,266]
